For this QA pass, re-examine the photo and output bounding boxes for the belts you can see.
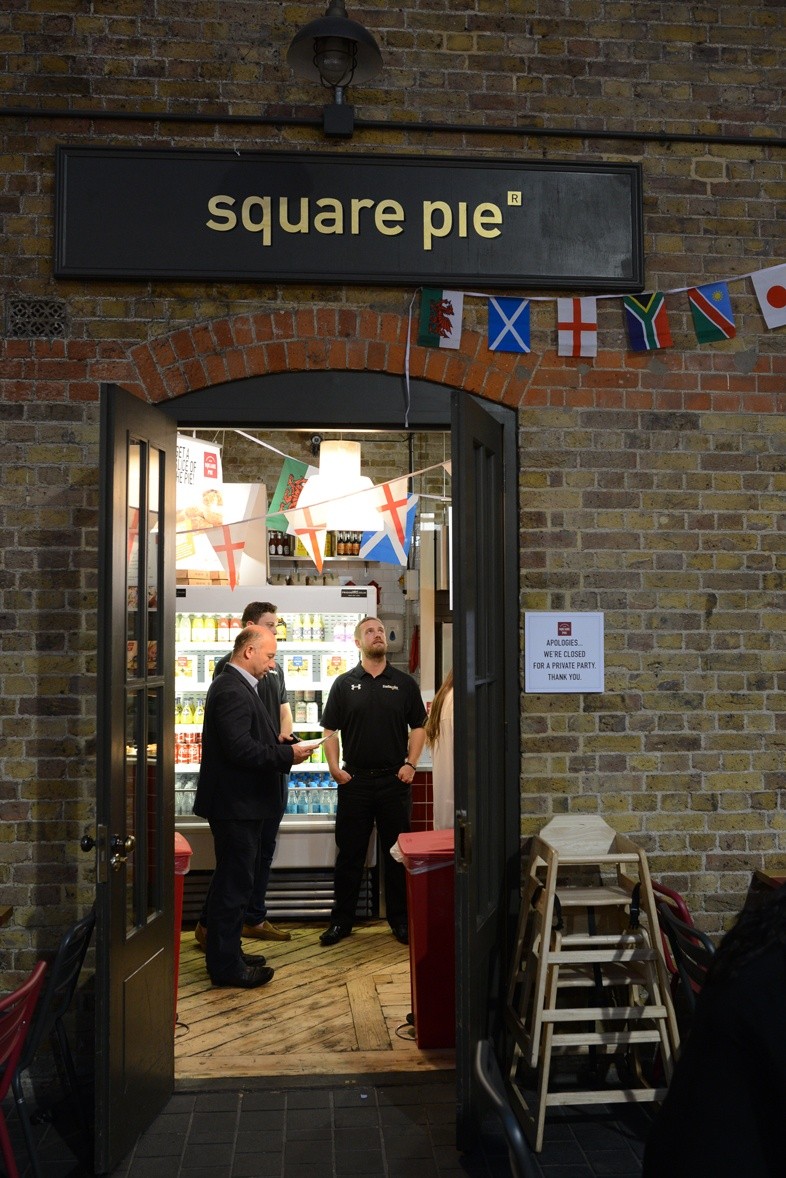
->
[344,762,404,777]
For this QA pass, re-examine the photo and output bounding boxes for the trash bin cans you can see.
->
[398,829,454,1049]
[174,831,193,1025]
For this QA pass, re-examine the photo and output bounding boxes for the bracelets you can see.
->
[405,761,416,772]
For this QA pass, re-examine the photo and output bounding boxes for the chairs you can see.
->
[0,958,50,1178]
[0,899,98,1178]
[474,811,720,1178]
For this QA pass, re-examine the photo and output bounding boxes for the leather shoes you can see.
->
[389,922,409,945]
[239,951,266,966]
[319,924,352,943]
[211,961,274,988]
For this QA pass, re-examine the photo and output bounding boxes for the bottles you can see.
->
[284,772,338,814]
[174,774,198,814]
[337,531,362,555]
[174,613,242,643]
[268,530,290,556]
[174,697,205,725]
[292,613,324,641]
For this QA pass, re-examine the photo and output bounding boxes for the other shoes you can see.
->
[195,922,207,953]
[242,919,291,941]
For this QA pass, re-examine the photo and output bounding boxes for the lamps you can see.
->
[283,0,386,139]
[287,439,384,533]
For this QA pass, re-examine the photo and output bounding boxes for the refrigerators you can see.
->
[173,584,379,923]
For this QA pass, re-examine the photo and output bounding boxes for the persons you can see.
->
[192,625,320,990]
[194,601,295,949]
[319,616,430,946]
[421,669,454,831]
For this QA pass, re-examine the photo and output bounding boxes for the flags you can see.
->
[360,488,419,567]
[623,292,673,354]
[751,263,786,330]
[487,294,532,354]
[687,281,738,343]
[264,456,318,537]
[416,287,463,351]
[556,296,599,360]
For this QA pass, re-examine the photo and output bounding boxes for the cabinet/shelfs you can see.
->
[176,585,379,920]
